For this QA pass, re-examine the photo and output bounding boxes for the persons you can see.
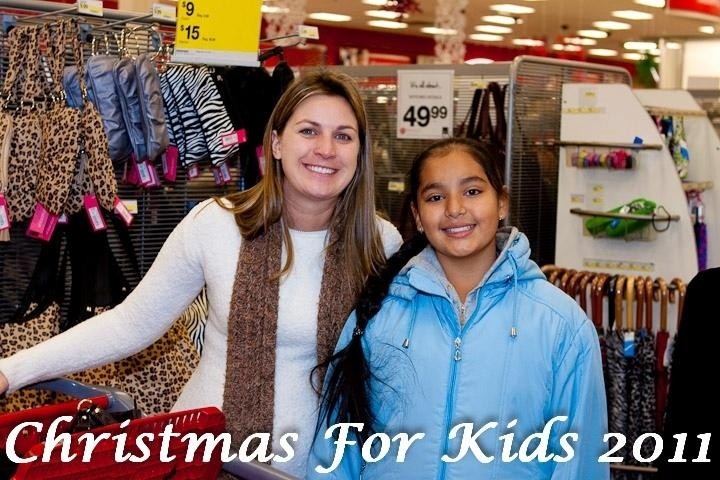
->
[298,137,611,480]
[1,66,407,479]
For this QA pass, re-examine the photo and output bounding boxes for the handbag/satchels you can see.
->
[0,301,202,417]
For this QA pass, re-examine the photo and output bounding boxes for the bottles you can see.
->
[694,204,708,272]
